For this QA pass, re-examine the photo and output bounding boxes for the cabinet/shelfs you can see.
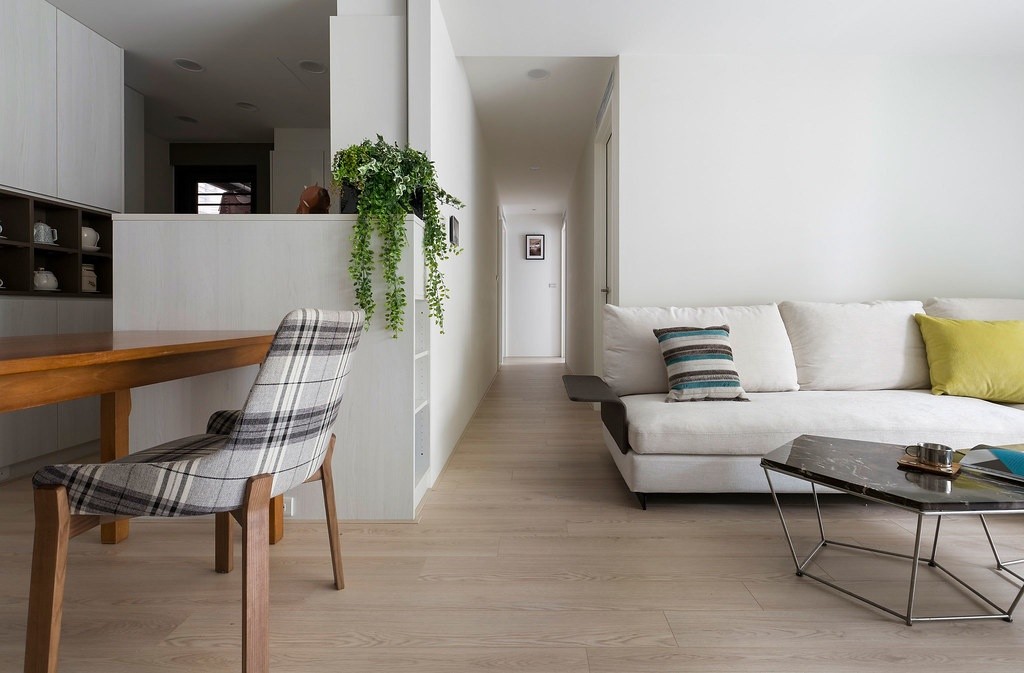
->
[266,0,409,211]
[110,213,432,522]
[0,0,128,298]
[0,297,112,481]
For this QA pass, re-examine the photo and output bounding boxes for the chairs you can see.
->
[23,307,365,673]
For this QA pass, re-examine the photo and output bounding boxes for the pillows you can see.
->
[914,313,1024,405]
[653,324,752,402]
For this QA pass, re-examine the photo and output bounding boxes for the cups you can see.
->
[905,443,953,468]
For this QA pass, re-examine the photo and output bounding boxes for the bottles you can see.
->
[82,264,97,290]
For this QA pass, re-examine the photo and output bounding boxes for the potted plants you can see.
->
[330,134,467,338]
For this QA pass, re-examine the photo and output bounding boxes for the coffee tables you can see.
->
[760,434,1024,625]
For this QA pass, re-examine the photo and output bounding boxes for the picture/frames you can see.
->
[526,235,545,260]
[450,215,460,246]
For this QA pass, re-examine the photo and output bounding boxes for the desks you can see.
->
[0,328,344,592]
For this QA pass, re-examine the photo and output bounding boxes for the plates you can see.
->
[34,242,59,246]
[81,290,101,293]
[82,247,101,251]
[0,236,8,240]
[33,289,62,291]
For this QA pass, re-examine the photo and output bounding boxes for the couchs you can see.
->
[563,296,1023,507]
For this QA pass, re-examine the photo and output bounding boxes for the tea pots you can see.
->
[34,220,57,243]
[82,227,99,246]
[34,268,58,288]
